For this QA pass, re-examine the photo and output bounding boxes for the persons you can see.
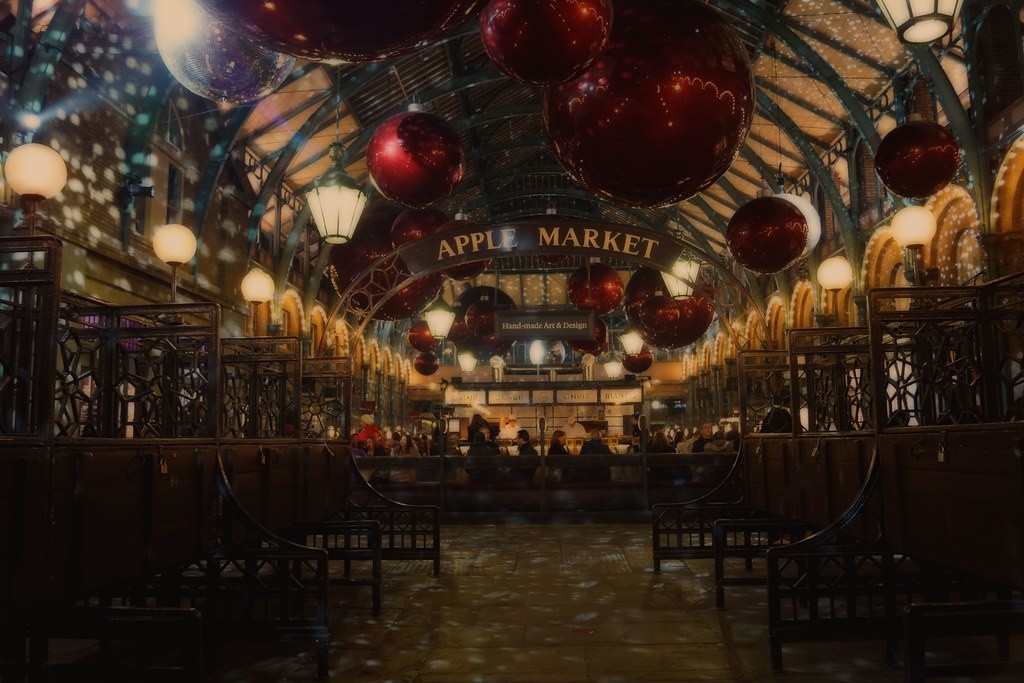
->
[627,414,741,454]
[560,414,587,439]
[349,413,386,457]
[579,429,615,455]
[547,429,570,457]
[466,413,502,457]
[388,430,429,458]
[498,414,523,440]
[758,395,794,434]
[515,429,539,456]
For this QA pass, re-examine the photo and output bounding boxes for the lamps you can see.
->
[424,286,456,341]
[816,256,851,328]
[305,63,372,246]
[457,355,477,375]
[154,223,196,327]
[4,144,67,271]
[240,266,275,339]
[877,0,963,45]
[603,332,623,381]
[891,205,937,311]
[659,231,700,302]
[615,320,645,357]
